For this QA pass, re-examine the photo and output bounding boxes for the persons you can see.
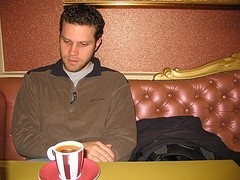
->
[11,4,137,163]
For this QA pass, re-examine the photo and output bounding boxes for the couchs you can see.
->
[0,52,240,160]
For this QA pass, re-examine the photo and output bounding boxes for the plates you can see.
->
[38,158,101,180]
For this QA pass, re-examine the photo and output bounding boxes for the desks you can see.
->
[0,160,240,180]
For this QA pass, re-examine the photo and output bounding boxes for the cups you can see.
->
[47,140,84,180]
